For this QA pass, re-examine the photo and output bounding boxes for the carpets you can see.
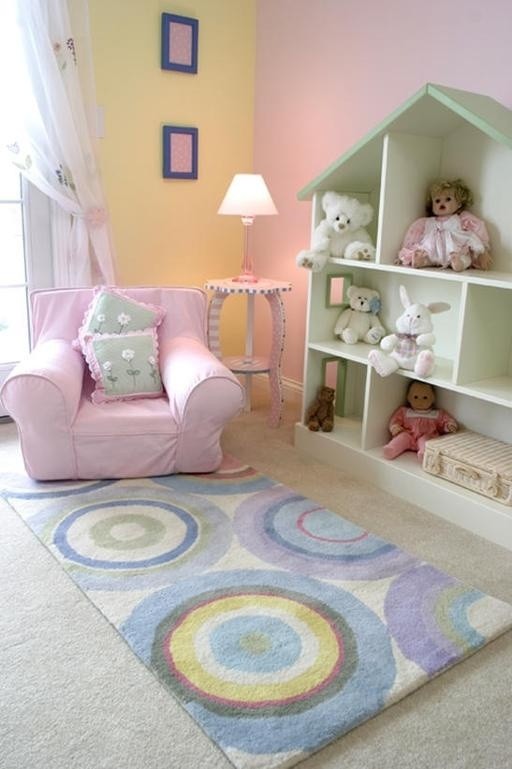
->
[0,453,511,769]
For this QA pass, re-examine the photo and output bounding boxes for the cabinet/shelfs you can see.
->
[293,84,510,548]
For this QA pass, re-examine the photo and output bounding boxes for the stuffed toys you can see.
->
[305,386,335,433]
[334,284,387,344]
[299,190,377,274]
[368,285,450,378]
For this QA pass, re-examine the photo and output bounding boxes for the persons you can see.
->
[382,383,459,463]
[395,180,496,273]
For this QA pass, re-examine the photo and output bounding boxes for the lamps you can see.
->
[216,172,281,284]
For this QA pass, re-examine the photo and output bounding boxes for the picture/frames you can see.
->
[162,12,199,76]
[161,125,200,182]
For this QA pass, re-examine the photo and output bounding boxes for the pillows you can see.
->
[72,287,164,357]
[81,327,165,404]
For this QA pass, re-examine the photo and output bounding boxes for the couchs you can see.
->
[0,285,244,482]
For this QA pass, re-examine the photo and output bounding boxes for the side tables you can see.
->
[202,276,293,429]
